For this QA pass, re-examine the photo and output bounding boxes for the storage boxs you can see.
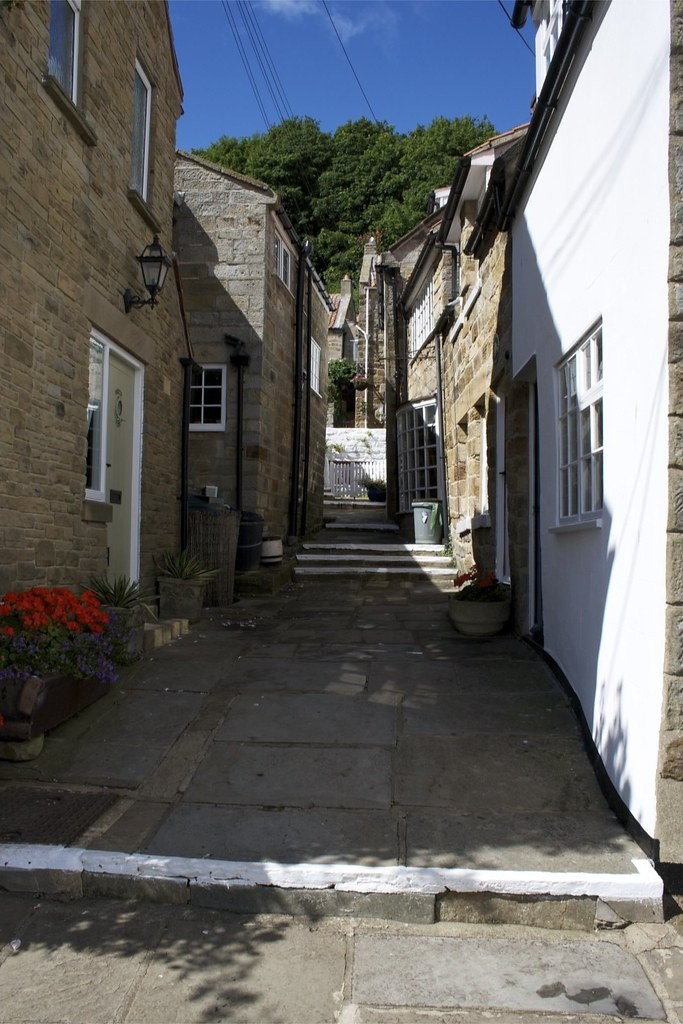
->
[205,486,218,498]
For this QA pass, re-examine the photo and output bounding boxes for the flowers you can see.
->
[0,586,145,689]
[350,372,372,385]
[452,562,508,603]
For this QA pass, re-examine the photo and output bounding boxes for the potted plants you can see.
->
[152,546,224,625]
[80,569,162,667]
[358,480,386,502]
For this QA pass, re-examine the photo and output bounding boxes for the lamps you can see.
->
[123,234,173,316]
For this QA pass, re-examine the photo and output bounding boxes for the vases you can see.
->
[410,501,443,544]
[448,595,512,638]
[259,536,284,567]
[0,673,111,740]
[353,382,369,391]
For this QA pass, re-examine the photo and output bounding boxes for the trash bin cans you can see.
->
[234,514,263,571]
[412,502,443,545]
